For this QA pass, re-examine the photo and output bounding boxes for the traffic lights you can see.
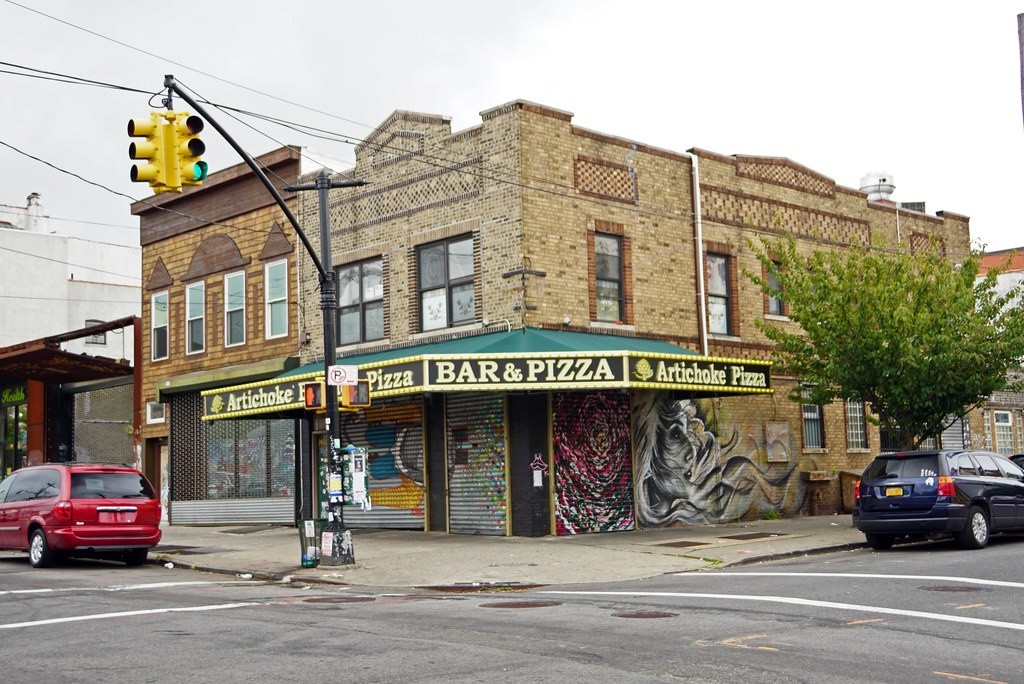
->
[303,380,330,410]
[170,114,209,188]
[128,111,180,194]
[345,380,371,409]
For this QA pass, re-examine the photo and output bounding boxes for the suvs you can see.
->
[851,449,1024,549]
[0,460,162,568]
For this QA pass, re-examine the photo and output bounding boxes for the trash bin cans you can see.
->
[297,518,327,568]
